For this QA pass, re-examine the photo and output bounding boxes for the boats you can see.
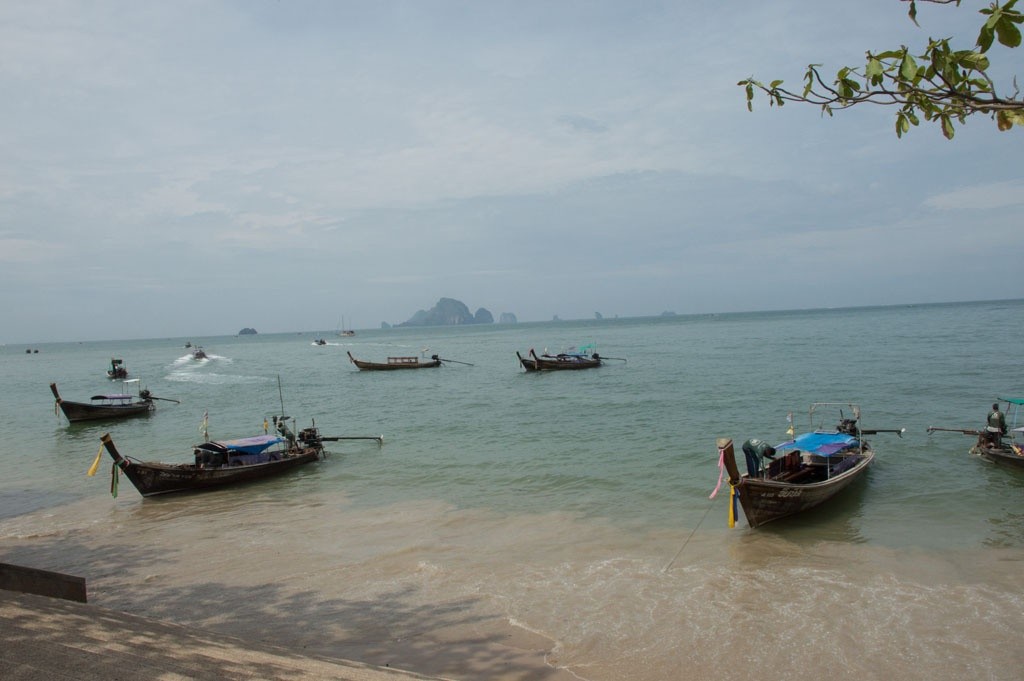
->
[716,403,876,531]
[516,348,601,370]
[106,360,128,377]
[314,338,326,346]
[24,349,39,355]
[968,427,1024,469]
[347,352,441,369]
[340,330,354,337]
[99,372,320,497]
[184,339,192,348]
[192,345,207,359]
[50,383,151,422]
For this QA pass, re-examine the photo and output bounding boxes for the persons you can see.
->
[983,403,1005,448]
[742,439,776,478]
[277,422,298,448]
